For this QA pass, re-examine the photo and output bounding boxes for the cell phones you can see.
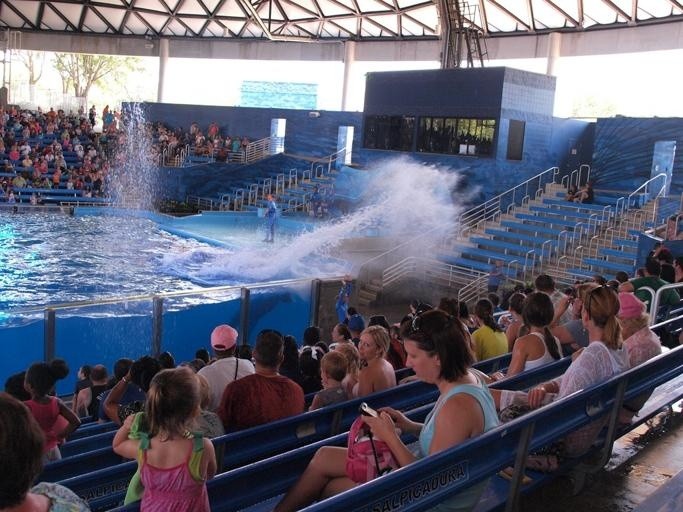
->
[358,402,380,418]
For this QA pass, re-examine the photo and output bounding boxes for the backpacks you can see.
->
[346,410,400,486]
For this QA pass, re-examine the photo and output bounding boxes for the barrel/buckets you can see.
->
[257,208,266,218]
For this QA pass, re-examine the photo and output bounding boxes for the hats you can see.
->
[210,323,239,352]
[616,291,646,319]
[347,313,366,331]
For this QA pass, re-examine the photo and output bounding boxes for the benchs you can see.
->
[434,190,682,286]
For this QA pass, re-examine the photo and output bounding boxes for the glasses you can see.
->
[589,284,619,321]
[412,311,432,340]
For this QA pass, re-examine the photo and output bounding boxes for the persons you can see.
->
[262,192,278,243]
[566,184,581,202]
[0,102,250,207]
[658,213,683,241]
[306,186,322,221]
[2,322,364,511]
[276,243,683,512]
[575,182,594,204]
